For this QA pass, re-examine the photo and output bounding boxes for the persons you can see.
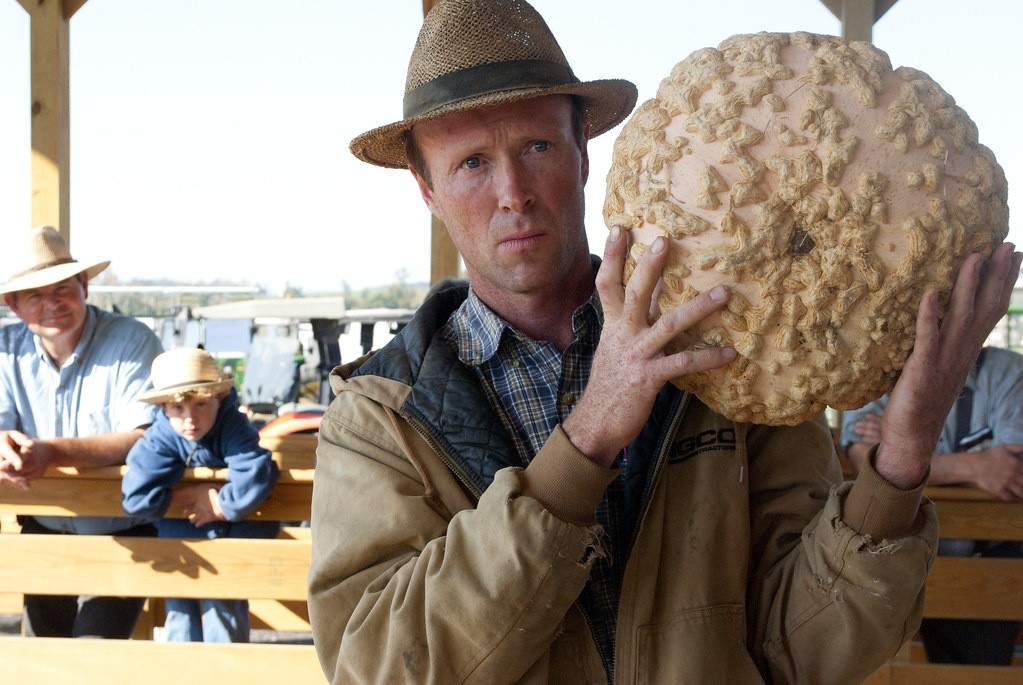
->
[120,346,281,643]
[0,225,166,641]
[306,0,1022,685]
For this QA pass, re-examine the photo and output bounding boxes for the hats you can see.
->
[137,347,235,402]
[0,225,111,307]
[349,0,639,169]
